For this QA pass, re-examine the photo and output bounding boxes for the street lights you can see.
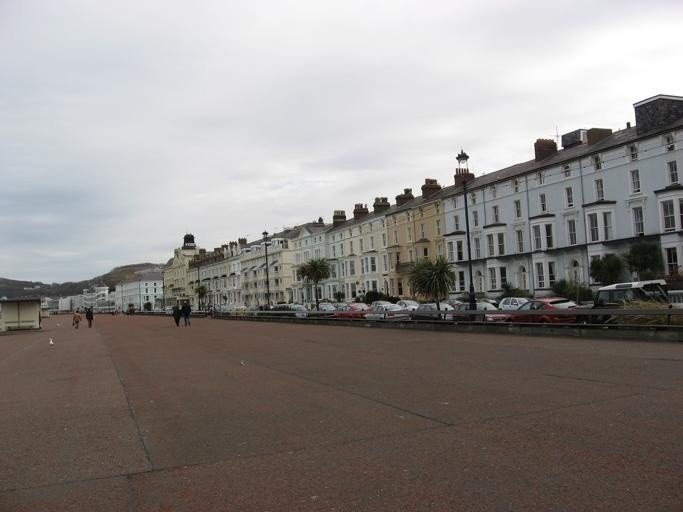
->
[455,149,476,321]
[262,230,269,310]
[196,257,200,311]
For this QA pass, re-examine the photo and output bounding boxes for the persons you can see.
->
[172,304,181,327]
[181,301,192,326]
[73,310,82,329]
[85,308,93,328]
